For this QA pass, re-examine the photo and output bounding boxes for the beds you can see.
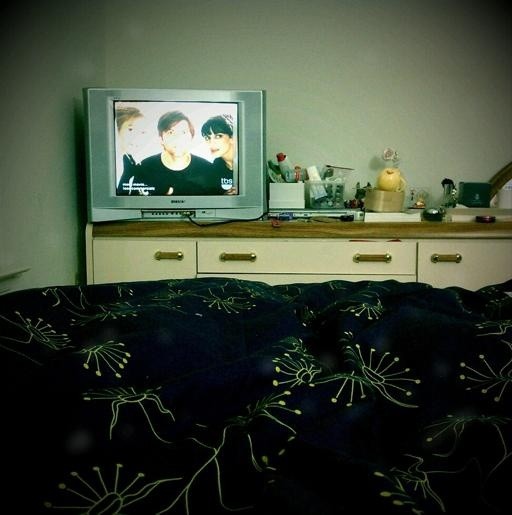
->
[1,274,511,514]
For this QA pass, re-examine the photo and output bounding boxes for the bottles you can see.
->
[440,178,454,206]
[275,151,297,184]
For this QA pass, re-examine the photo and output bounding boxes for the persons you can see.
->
[114,107,238,196]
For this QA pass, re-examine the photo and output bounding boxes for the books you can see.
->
[363,209,422,223]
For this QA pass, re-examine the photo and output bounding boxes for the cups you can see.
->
[377,167,407,193]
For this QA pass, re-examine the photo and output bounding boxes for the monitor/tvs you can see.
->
[82,87,268,223]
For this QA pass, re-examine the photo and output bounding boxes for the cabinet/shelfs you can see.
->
[84,222,512,291]
